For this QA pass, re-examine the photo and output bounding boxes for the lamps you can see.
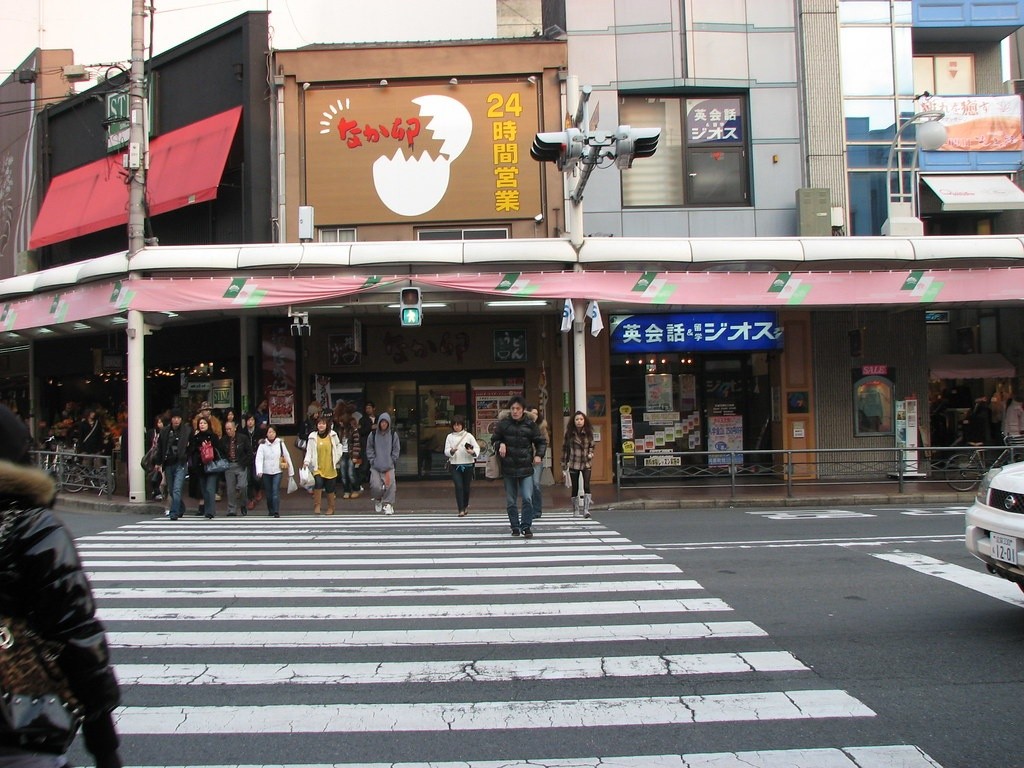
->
[880,111,946,236]
[527,75,536,85]
[449,78,458,85]
[303,83,311,91]
[380,79,388,86]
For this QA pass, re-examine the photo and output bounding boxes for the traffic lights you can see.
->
[400,288,422,327]
[530,128,583,172]
[617,125,662,170]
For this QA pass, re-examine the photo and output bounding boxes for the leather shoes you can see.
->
[241,506,247,515]
[226,513,237,516]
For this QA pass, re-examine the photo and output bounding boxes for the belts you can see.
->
[230,460,237,462]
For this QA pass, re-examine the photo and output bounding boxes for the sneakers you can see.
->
[255,491,263,504]
[268,512,279,518]
[248,500,254,510]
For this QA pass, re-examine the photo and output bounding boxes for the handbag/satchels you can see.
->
[279,441,289,470]
[0,499,85,755]
[141,446,158,472]
[562,466,572,489]
[299,466,315,486]
[485,442,502,478]
[294,423,308,451]
[443,458,450,473]
[287,477,298,494]
[199,440,231,473]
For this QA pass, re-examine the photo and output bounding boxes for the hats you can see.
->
[198,401,213,410]
[307,405,318,416]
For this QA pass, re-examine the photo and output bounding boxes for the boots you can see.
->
[194,505,205,516]
[571,496,579,517]
[584,494,591,518]
[325,492,335,515]
[314,489,322,514]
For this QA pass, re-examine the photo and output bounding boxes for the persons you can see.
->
[192,418,221,519]
[445,416,480,517]
[120,400,269,516]
[367,413,401,515]
[36,419,57,451]
[300,400,378,500]
[255,426,294,518]
[76,406,103,491]
[303,418,343,515]
[560,412,595,519]
[156,410,192,521]
[491,397,549,538]
[0,402,124,768]
[220,422,252,517]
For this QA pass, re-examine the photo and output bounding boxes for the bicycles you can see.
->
[39,436,117,497]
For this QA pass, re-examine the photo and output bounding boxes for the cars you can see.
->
[944,432,1022,492]
[965,462,1024,594]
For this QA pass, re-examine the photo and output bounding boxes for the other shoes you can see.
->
[171,513,183,520]
[374,500,395,515]
[459,508,468,517]
[343,491,359,498]
[511,527,533,536]
